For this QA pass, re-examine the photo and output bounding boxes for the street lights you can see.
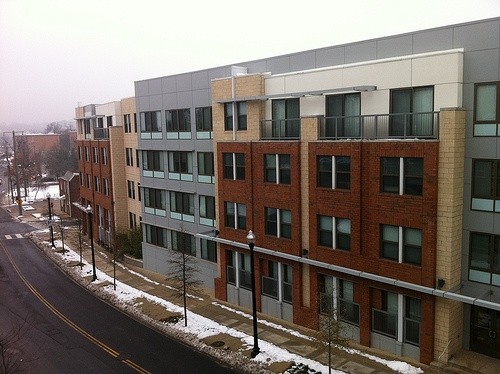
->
[246,229,260,358]
[86,204,98,282]
[46,192,55,249]
